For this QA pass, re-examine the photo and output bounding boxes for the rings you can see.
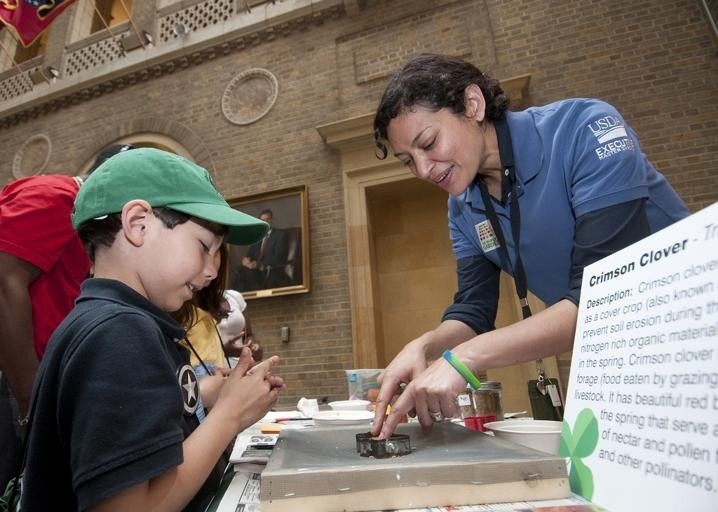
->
[429,410,442,422]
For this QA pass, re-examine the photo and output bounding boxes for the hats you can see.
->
[70,148,270,246]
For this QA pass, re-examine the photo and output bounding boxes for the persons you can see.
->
[371,53,692,440]
[230,211,287,292]
[18,148,287,511]
[1,148,129,497]
[175,241,263,379]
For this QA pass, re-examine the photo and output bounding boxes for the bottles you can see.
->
[457,379,503,420]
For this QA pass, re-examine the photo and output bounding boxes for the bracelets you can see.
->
[442,349,481,391]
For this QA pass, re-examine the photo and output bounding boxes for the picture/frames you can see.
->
[222,185,310,301]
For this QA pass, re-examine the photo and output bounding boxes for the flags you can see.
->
[0,0,77,48]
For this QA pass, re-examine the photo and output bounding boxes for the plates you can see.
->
[314,398,375,425]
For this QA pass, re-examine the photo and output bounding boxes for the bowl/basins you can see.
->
[483,416,563,455]
